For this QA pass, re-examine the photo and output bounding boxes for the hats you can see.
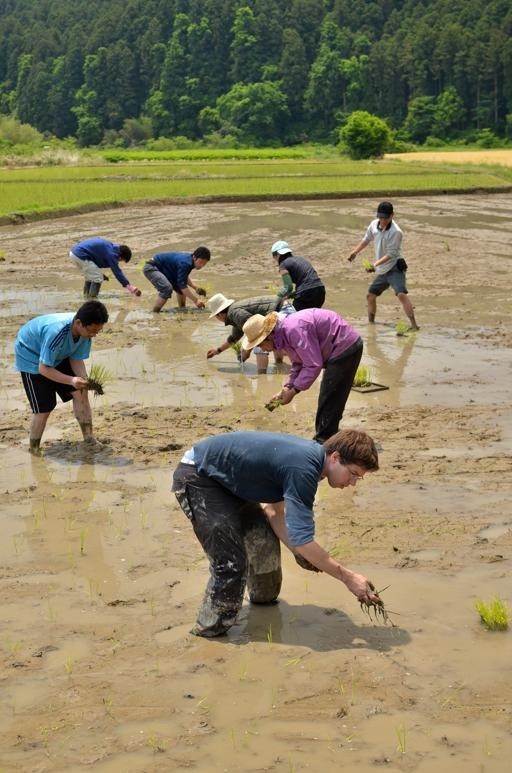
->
[376,202,393,219]
[242,311,279,351]
[272,240,293,255]
[207,292,235,319]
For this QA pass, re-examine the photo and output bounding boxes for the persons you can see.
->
[12,300,110,454]
[205,292,296,373]
[348,200,418,331]
[240,307,363,447]
[68,237,141,298]
[269,239,326,313]
[168,428,380,640]
[142,245,210,314]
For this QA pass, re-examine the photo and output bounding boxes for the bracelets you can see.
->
[218,348,222,353]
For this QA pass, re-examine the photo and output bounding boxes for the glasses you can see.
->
[85,326,103,335]
[342,459,363,481]
